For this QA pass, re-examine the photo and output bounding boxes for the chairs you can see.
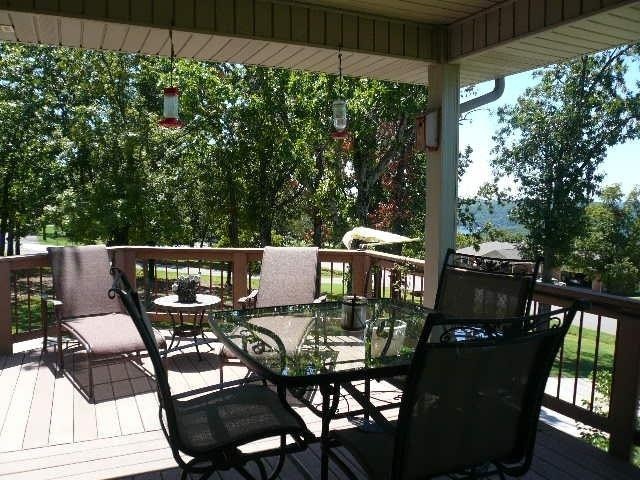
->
[43,245,170,401]
[327,304,582,478]
[216,246,327,389]
[108,266,310,478]
[371,249,548,424]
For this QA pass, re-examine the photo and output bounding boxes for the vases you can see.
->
[177,289,196,302]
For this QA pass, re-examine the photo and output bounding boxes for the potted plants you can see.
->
[363,248,427,357]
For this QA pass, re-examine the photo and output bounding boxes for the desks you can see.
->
[208,297,434,478]
[153,294,223,361]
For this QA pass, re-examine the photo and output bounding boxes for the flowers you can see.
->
[171,273,203,292]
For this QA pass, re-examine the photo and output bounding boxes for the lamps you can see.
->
[156,28,183,129]
[330,47,349,139]
